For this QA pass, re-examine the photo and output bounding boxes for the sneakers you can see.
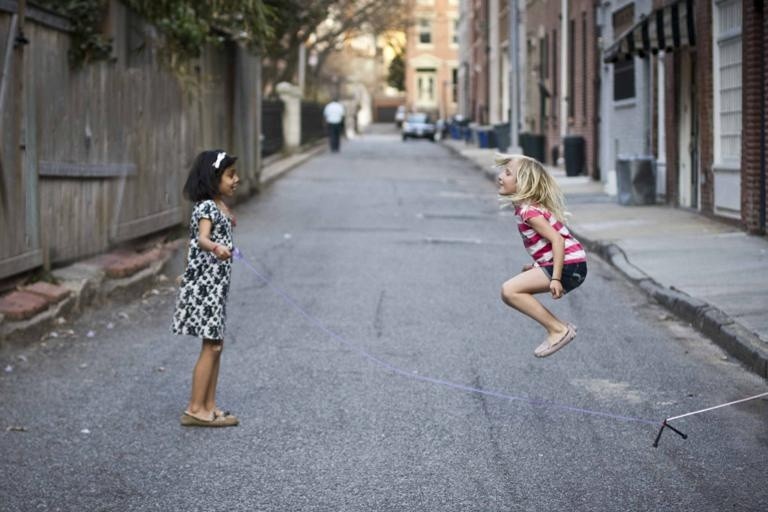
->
[180,413,224,428]
[534,326,576,357]
[565,323,577,332]
[218,413,239,425]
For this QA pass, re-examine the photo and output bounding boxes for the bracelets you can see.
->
[212,244,219,252]
[550,279,561,282]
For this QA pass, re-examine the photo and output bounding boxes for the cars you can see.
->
[400,111,438,144]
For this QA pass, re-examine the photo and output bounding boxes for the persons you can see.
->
[322,97,345,152]
[170,149,243,426]
[490,152,588,359]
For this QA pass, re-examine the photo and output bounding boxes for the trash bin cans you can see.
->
[519,133,545,163]
[615,157,654,206]
[563,136,584,176]
[494,123,509,151]
